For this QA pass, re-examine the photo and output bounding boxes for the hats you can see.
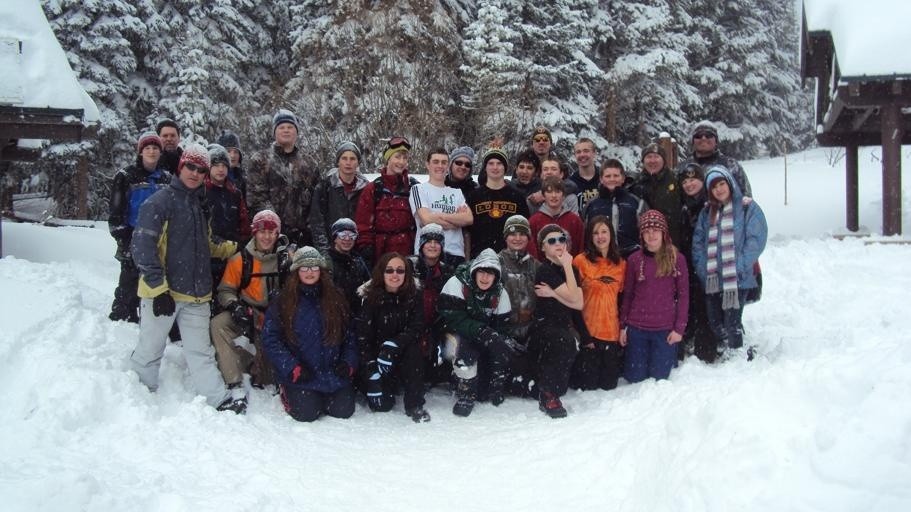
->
[678,165,704,185]
[272,109,300,137]
[706,172,726,191]
[138,131,164,154]
[482,148,509,173]
[641,144,668,169]
[419,222,445,261]
[330,217,359,241]
[532,128,552,143]
[537,224,566,248]
[503,215,531,240]
[692,124,718,144]
[206,143,232,180]
[251,210,281,235]
[639,209,670,248]
[178,144,211,179]
[290,245,328,272]
[216,130,242,164]
[448,146,474,180]
[335,141,362,164]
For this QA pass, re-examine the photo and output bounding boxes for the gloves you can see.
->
[367,360,385,409]
[334,361,354,376]
[153,290,176,316]
[288,364,314,384]
[377,341,400,376]
[227,300,249,327]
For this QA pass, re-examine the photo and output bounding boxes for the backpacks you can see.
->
[737,197,762,304]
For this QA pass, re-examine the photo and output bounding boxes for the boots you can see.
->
[453,374,479,417]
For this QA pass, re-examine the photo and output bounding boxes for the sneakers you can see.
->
[539,391,567,418]
[406,404,430,423]
[216,397,246,416]
[227,381,249,414]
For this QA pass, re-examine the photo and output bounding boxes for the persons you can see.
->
[527,157,579,216]
[571,138,601,214]
[528,176,584,263]
[583,159,651,261]
[246,109,323,244]
[678,120,753,199]
[692,165,768,363]
[511,152,577,214]
[354,251,431,424]
[619,209,688,385]
[533,223,584,419]
[409,148,474,267]
[107,131,173,325]
[498,214,543,334]
[216,132,246,204]
[206,144,251,319]
[262,246,361,422]
[436,246,513,418]
[355,135,422,268]
[572,212,627,391]
[127,142,230,411]
[156,120,182,175]
[445,145,478,201]
[462,149,530,262]
[679,163,718,364]
[309,142,369,257]
[211,209,294,416]
[406,223,454,390]
[626,143,684,244]
[319,219,373,304]
[531,126,559,163]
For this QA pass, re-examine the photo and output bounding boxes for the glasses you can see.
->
[299,266,320,272]
[454,160,472,168]
[184,163,207,174]
[693,133,714,138]
[420,232,445,240]
[544,236,568,245]
[383,143,409,166]
[385,268,405,274]
[383,137,411,156]
[335,231,358,241]
[533,135,550,142]
